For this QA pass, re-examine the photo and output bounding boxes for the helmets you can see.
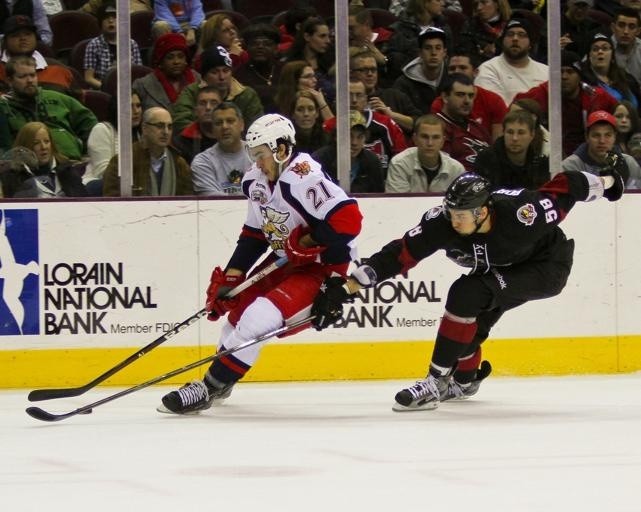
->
[244,112,298,164]
[441,172,491,225]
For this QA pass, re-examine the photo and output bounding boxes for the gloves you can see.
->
[282,222,325,267]
[204,265,245,321]
[311,275,354,331]
[601,147,630,203]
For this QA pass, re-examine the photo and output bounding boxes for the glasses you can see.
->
[591,44,612,51]
[299,73,318,80]
[353,66,377,74]
[506,30,525,37]
[145,121,173,129]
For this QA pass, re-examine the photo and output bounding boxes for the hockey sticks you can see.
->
[25,314,327,422]
[27,255,288,401]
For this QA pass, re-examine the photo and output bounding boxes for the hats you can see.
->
[586,109,619,132]
[199,45,232,75]
[589,31,613,45]
[505,16,532,32]
[350,107,368,131]
[417,25,447,47]
[96,4,115,20]
[561,48,584,72]
[4,13,37,34]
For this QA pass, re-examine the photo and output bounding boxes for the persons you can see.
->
[0,1,641,198]
[310,168,625,407]
[160,112,365,416]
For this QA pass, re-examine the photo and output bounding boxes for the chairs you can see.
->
[0,1,641,195]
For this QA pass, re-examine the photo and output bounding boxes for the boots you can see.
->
[395,358,458,407]
[441,359,493,403]
[161,380,230,412]
